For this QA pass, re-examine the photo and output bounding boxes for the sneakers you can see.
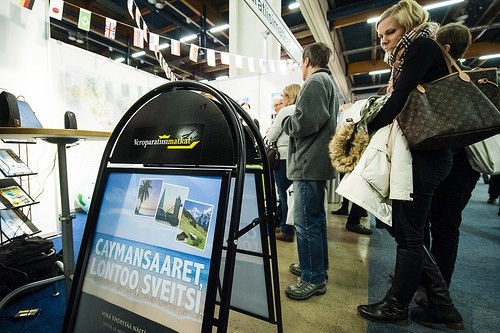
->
[290,263,328,282]
[285,276,327,300]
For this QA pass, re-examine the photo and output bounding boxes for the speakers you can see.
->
[64,111,77,129]
[0,91,21,126]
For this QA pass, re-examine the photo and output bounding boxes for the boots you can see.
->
[357,249,421,322]
[332,202,349,215]
[407,246,465,331]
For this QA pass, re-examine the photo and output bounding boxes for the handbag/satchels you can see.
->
[392,38,499,152]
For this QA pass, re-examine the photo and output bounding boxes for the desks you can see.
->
[0,126,112,313]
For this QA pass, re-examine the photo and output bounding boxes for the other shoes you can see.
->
[275,227,282,232]
[346,222,372,235]
[275,233,294,242]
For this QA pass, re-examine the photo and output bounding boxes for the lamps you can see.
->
[68,31,84,44]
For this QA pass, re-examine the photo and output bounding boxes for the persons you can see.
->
[267,0,500,329]
[241,103,260,147]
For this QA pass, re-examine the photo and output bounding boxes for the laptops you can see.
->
[17,100,43,128]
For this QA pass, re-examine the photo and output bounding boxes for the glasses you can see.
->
[272,103,283,109]
[280,94,289,97]
